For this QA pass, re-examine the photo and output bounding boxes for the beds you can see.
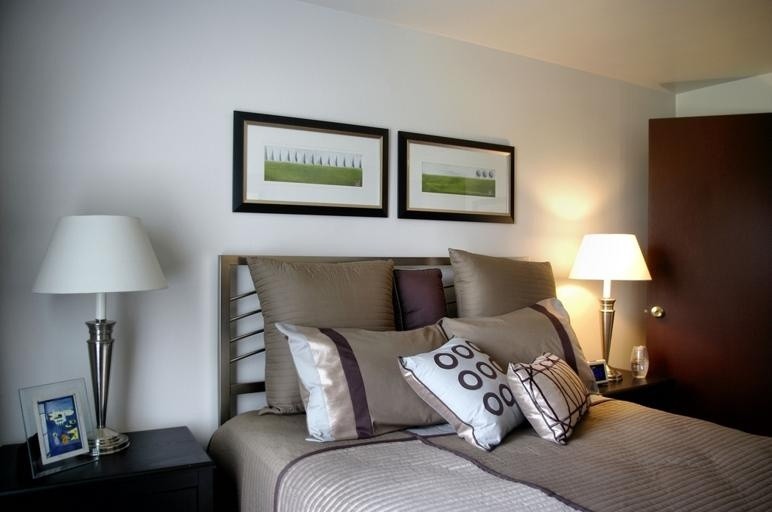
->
[207,254,772,512]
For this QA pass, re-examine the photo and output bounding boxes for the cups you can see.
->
[630,345,650,380]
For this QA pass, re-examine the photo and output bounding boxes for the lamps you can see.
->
[569,233,653,383]
[31,214,169,457]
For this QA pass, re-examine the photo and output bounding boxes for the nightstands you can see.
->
[598,367,656,404]
[0,426,217,512]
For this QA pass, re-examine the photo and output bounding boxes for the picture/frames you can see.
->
[18,378,100,479]
[232,110,389,219]
[397,130,515,224]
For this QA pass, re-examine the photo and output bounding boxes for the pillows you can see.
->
[245,256,395,417]
[393,268,448,331]
[397,334,525,453]
[448,248,556,319]
[507,350,592,446]
[441,297,597,388]
[274,321,450,443]
